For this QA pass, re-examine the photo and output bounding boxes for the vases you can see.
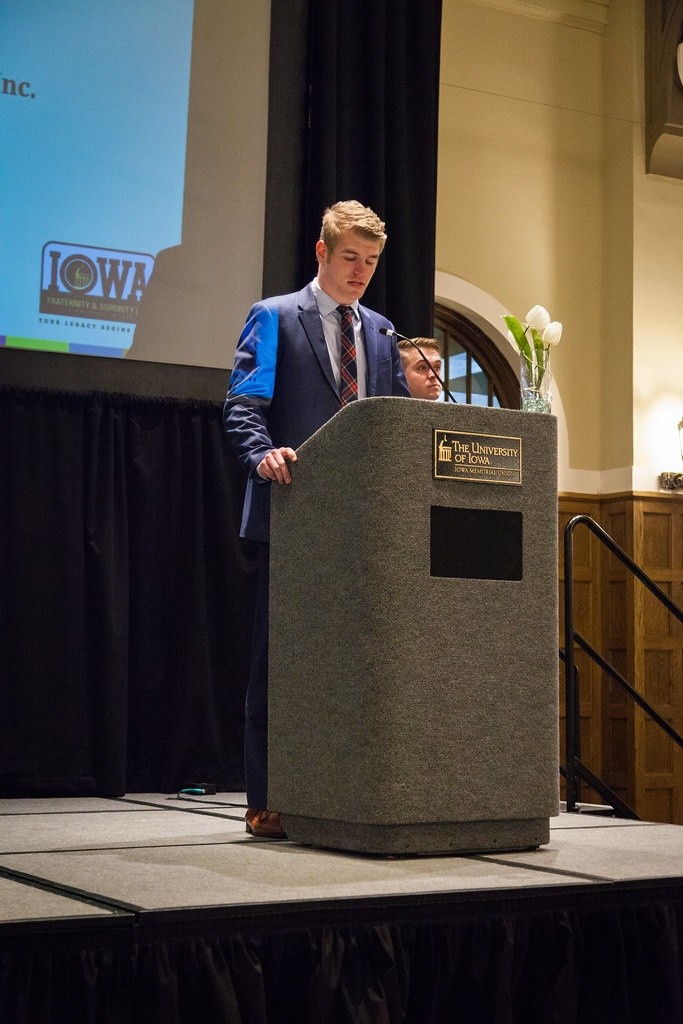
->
[519,345,552,415]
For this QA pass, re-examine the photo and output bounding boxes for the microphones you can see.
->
[379,327,457,404]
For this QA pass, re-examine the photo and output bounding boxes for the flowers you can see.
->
[501,305,562,401]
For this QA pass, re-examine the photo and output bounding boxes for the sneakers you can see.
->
[245,807,285,838]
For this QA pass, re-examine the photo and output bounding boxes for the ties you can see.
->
[336,305,358,408]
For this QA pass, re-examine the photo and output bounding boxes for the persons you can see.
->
[223,200,411,837]
[397,337,442,401]
